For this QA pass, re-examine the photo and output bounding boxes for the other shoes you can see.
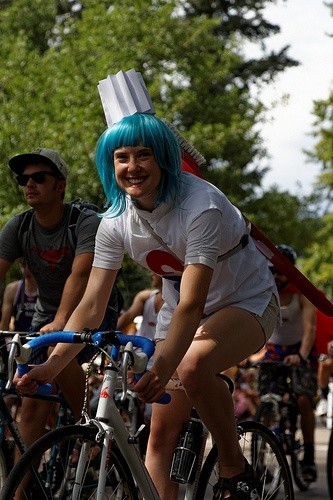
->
[301,461,317,482]
[212,457,253,500]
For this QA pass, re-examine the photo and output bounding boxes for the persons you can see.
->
[0,113,333,500]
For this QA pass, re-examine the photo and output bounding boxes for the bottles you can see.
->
[279,407,292,449]
[169,409,203,486]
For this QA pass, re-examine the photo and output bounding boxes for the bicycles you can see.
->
[0,328,333,500]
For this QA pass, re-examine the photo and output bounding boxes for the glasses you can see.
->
[270,269,284,275]
[16,171,58,186]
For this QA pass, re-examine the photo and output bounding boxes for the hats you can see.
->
[8,148,68,180]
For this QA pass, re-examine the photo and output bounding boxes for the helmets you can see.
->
[277,245,297,263]
